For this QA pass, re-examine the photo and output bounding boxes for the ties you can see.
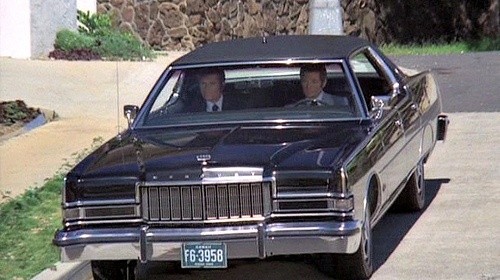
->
[211,104,218,112]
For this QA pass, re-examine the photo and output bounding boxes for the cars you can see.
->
[51,29,448,279]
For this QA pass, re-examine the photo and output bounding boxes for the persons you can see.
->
[178,66,249,115]
[283,64,353,113]
